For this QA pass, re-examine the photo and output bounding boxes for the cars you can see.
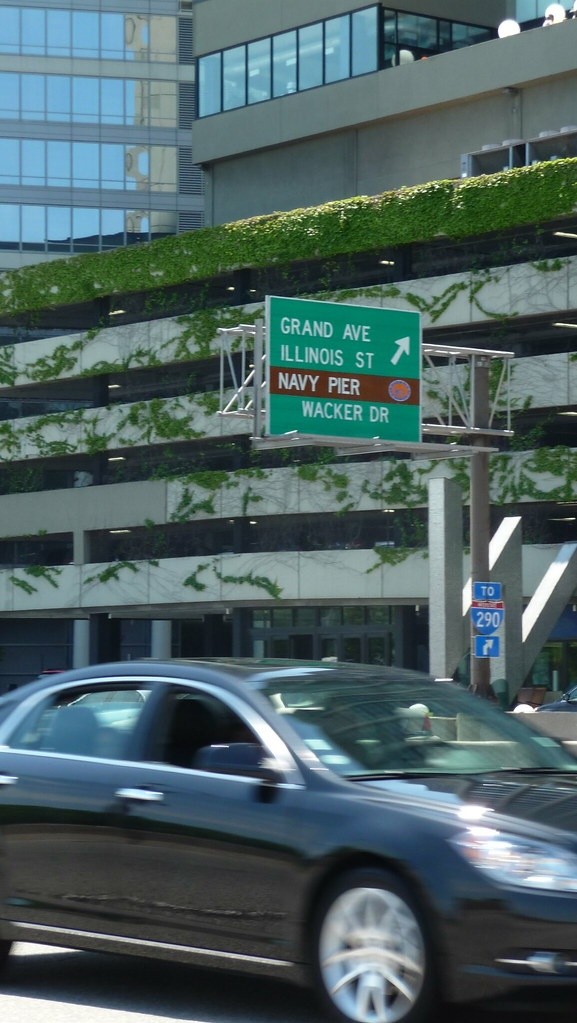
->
[0,656,577,1022]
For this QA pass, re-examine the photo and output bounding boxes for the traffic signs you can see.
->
[265,296,424,447]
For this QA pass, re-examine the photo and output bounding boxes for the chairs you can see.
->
[162,691,242,771]
[46,706,103,757]
